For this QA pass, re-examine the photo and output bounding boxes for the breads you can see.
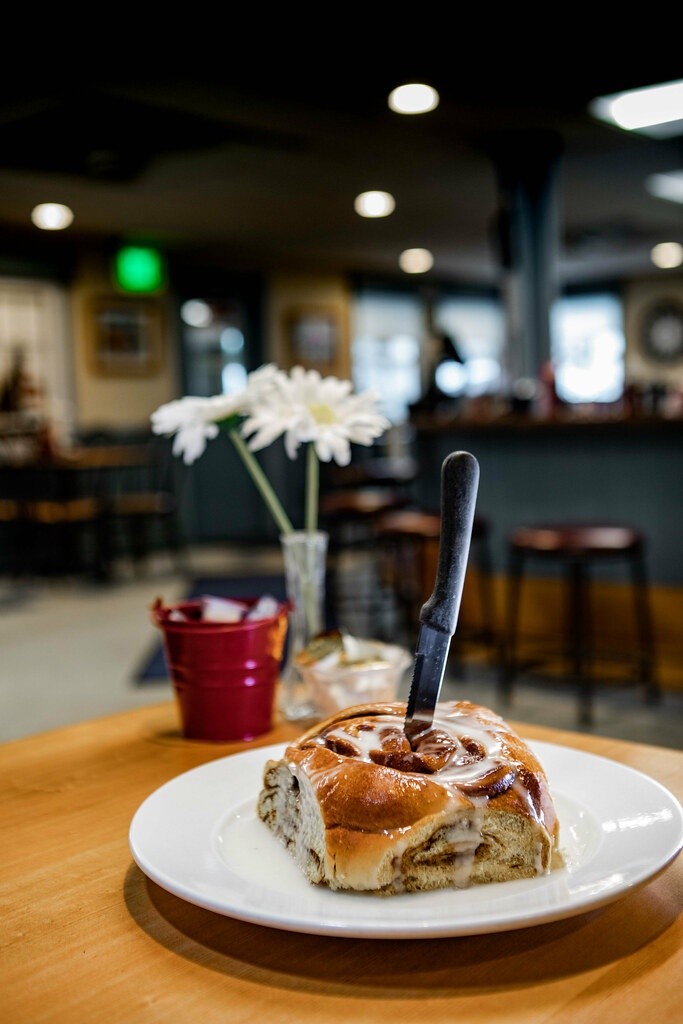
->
[258,699,558,893]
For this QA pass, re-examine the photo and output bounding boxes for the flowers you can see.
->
[149,362,391,638]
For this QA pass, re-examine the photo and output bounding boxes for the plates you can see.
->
[129,736,683,938]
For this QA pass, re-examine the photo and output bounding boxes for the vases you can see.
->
[279,532,332,723]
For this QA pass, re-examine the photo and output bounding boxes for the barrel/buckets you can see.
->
[151,596,294,743]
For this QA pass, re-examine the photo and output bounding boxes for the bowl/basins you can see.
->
[291,642,413,719]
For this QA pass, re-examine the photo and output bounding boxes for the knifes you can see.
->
[404,452,479,739]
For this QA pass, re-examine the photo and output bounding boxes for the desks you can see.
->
[0,686,683,1024]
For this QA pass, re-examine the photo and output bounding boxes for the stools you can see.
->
[318,489,424,638]
[503,520,673,731]
[377,512,495,688]
